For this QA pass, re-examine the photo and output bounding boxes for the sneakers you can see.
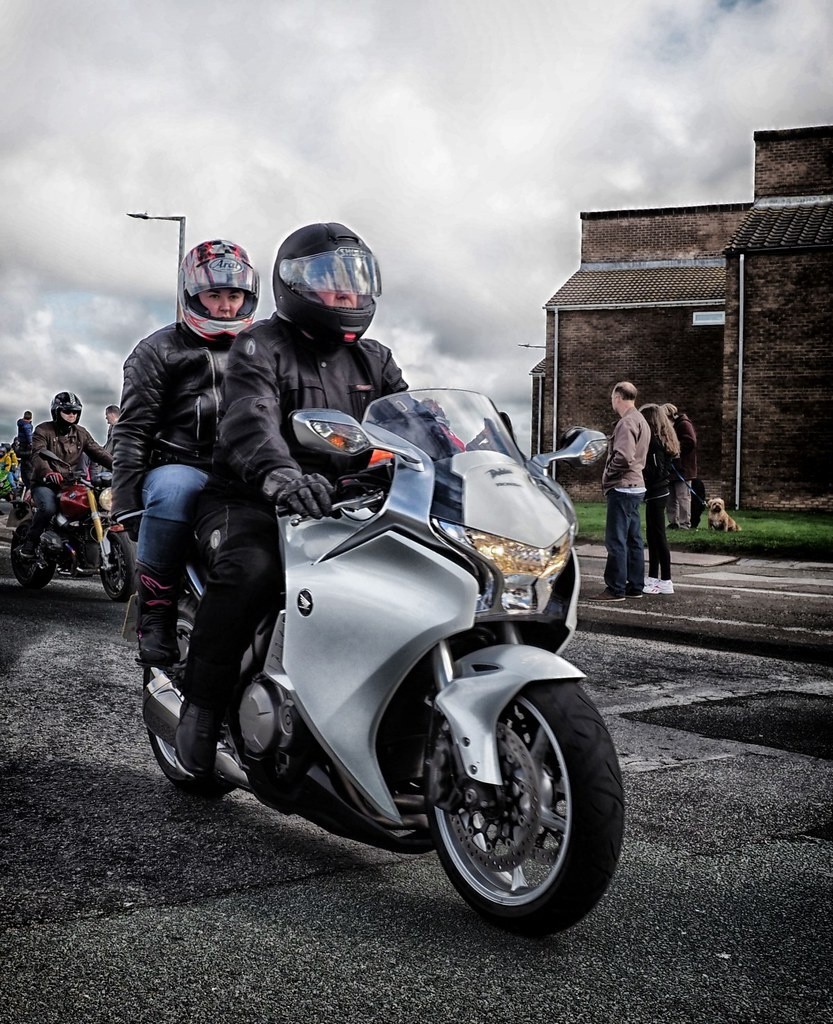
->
[587,589,626,601]
[643,580,674,595]
[20,543,34,558]
[625,583,643,598]
[645,577,658,584]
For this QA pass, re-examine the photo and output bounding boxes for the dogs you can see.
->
[706,497,743,532]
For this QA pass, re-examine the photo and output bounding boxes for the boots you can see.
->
[134,559,182,667]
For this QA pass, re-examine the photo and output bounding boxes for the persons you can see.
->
[109,238,261,666]
[466,412,528,462]
[0,405,119,515]
[173,222,461,781]
[587,382,651,602]
[662,403,698,530]
[421,398,465,452]
[639,403,678,595]
[18,391,114,558]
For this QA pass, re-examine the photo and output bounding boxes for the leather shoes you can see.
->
[173,698,221,777]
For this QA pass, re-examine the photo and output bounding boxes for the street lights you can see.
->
[126,213,187,322]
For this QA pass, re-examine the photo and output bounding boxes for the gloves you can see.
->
[263,467,343,521]
[45,472,63,485]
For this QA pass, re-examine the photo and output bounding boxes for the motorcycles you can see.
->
[120,390,625,941]
[0,462,15,503]
[6,447,138,604]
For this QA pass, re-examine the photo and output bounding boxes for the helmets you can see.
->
[0,446,7,456]
[50,391,82,422]
[273,222,382,347]
[180,241,259,342]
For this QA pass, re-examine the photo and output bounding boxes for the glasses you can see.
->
[62,409,77,415]
